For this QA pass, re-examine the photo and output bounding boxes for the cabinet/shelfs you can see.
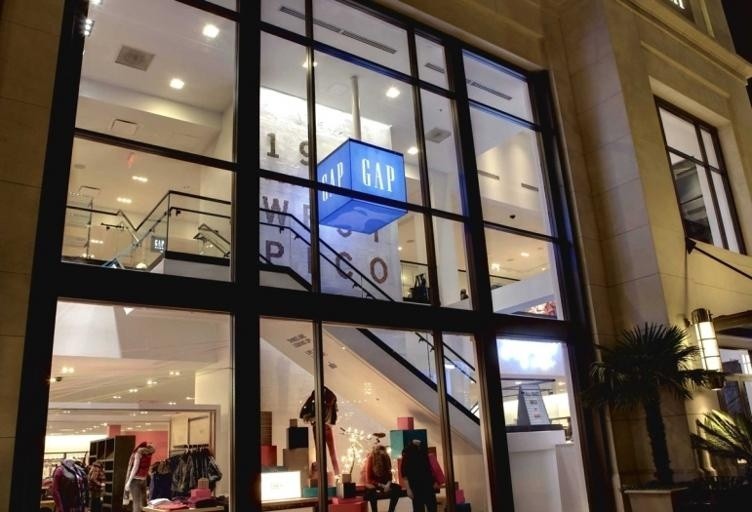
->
[89,435,136,512]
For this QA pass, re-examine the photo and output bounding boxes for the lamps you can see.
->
[686,309,725,390]
[317,74,409,233]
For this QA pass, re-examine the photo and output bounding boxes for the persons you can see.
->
[51,460,106,512]
[300,385,342,479]
[401,437,440,512]
[123,442,155,512]
[363,446,401,512]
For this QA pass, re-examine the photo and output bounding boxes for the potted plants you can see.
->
[577,317,738,512]
